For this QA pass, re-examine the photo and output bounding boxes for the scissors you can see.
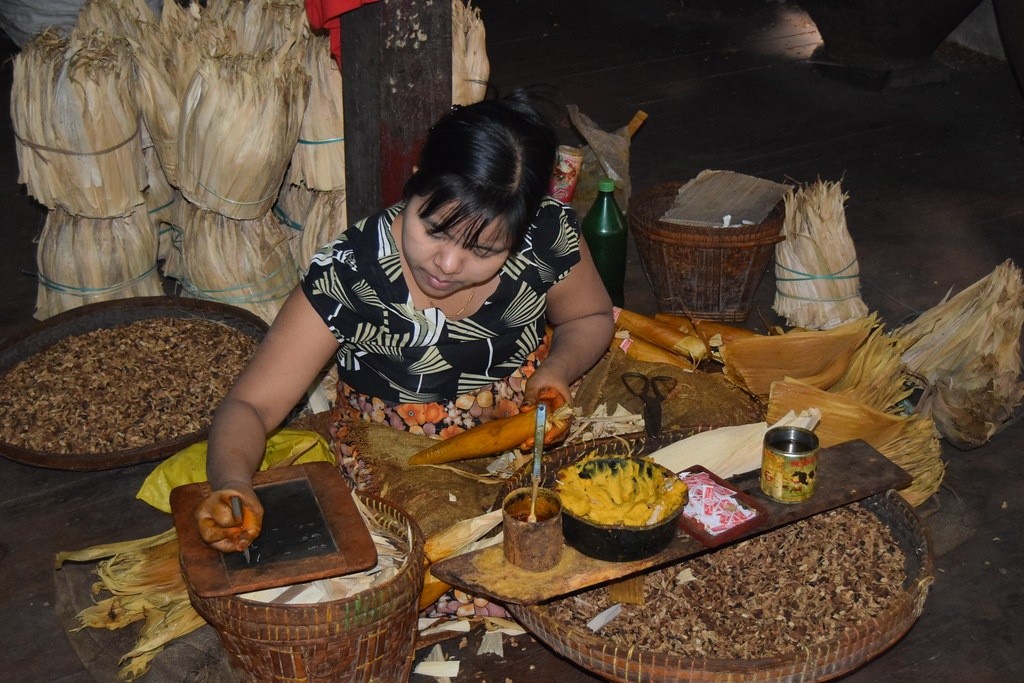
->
[620,372,678,438]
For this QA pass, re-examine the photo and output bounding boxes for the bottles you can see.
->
[582,180,628,309]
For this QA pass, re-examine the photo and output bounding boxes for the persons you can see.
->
[194,101,615,555]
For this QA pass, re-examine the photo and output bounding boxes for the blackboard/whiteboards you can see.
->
[169,459,380,599]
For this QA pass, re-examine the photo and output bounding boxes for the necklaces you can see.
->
[428,286,476,319]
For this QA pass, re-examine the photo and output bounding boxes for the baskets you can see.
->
[0,294,271,471]
[627,181,787,324]
[489,423,936,682]
[178,487,428,683]
